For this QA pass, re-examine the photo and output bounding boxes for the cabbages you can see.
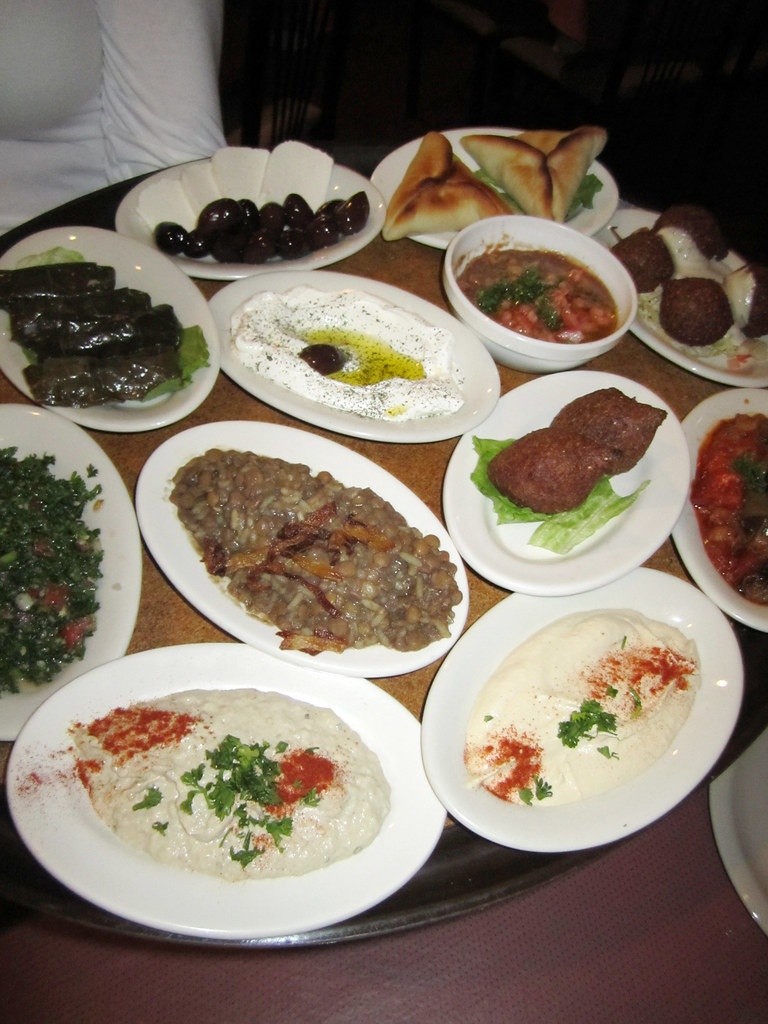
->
[8,247,211,402]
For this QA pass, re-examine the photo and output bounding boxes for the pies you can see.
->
[459,125,608,224]
[381,132,515,242]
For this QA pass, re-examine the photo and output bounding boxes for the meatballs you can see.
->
[610,207,768,347]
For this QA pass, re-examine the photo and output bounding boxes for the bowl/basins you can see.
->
[442,215,638,374]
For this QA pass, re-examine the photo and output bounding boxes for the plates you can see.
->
[136,420,469,679]
[113,159,386,280]
[370,124,619,250]
[0,227,220,434]
[420,569,745,854]
[592,206,767,388]
[670,389,768,633]
[0,403,142,742]
[707,725,767,938]
[208,270,502,445]
[8,641,446,940]
[441,369,690,597]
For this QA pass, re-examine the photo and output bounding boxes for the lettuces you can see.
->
[467,432,652,557]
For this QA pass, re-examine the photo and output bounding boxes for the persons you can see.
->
[0,0,229,243]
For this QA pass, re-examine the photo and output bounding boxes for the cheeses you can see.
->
[136,138,335,234]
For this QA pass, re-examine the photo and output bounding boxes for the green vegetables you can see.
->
[132,736,321,874]
[0,446,103,696]
[484,635,645,806]
[470,164,604,226]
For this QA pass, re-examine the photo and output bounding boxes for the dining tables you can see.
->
[0,154,768,1024]
